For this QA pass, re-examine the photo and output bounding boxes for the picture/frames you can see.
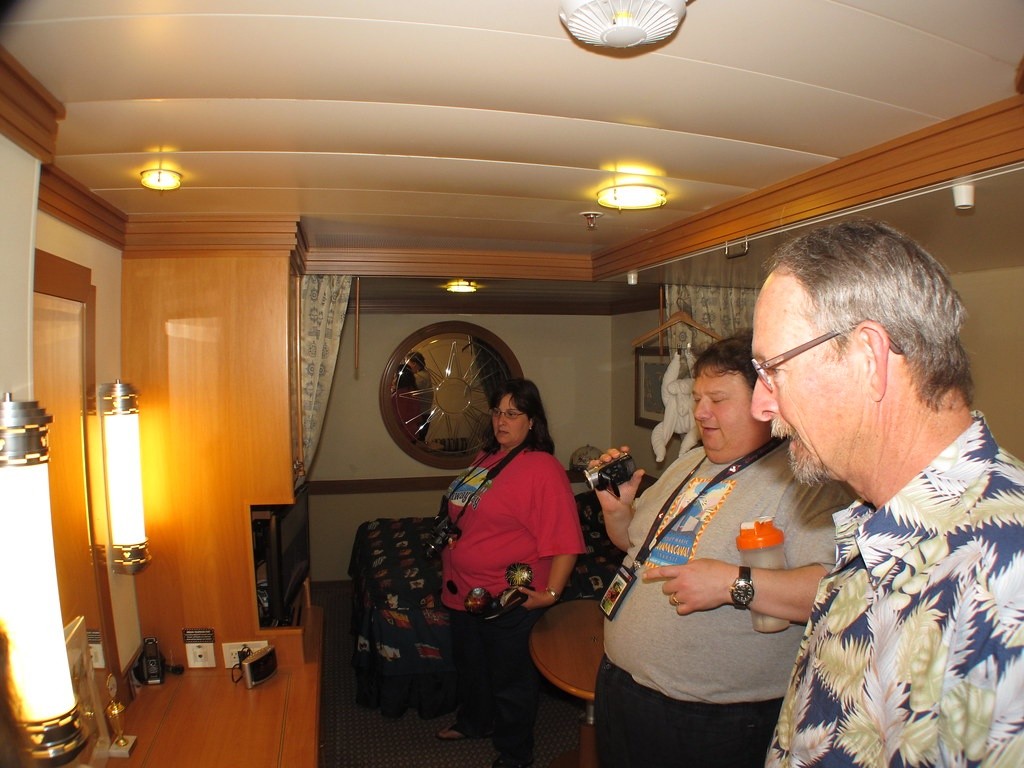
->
[632,345,700,438]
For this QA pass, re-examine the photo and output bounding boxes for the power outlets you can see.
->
[222,641,268,669]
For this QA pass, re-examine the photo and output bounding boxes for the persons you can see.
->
[588,335,856,768]
[394,364,423,439]
[406,352,434,441]
[751,219,1024,768]
[440,378,587,768]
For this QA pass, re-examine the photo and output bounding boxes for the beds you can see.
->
[347,489,623,714]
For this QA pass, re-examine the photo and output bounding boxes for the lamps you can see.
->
[597,185,670,210]
[627,272,639,285]
[953,184,976,210]
[0,393,91,767]
[102,380,153,573]
[139,170,181,191]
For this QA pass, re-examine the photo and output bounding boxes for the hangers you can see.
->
[639,301,728,344]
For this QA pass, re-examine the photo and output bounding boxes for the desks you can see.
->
[530,602,611,768]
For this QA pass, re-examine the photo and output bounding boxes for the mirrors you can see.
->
[34,249,128,743]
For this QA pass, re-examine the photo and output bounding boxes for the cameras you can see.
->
[242,645,277,689]
[426,512,461,552]
[582,452,636,499]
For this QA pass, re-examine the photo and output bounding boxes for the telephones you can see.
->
[131,636,166,685]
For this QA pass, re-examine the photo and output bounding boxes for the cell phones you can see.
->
[143,637,159,679]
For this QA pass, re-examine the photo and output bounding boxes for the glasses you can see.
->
[488,406,526,419]
[751,325,903,392]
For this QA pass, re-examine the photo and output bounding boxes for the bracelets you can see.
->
[545,587,559,600]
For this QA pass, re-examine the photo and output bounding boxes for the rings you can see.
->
[673,595,680,604]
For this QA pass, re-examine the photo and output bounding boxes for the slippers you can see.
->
[492,753,537,768]
[442,722,496,740]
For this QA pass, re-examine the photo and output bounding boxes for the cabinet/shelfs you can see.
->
[123,212,310,487]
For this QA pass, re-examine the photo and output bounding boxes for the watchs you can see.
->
[730,566,754,610]
